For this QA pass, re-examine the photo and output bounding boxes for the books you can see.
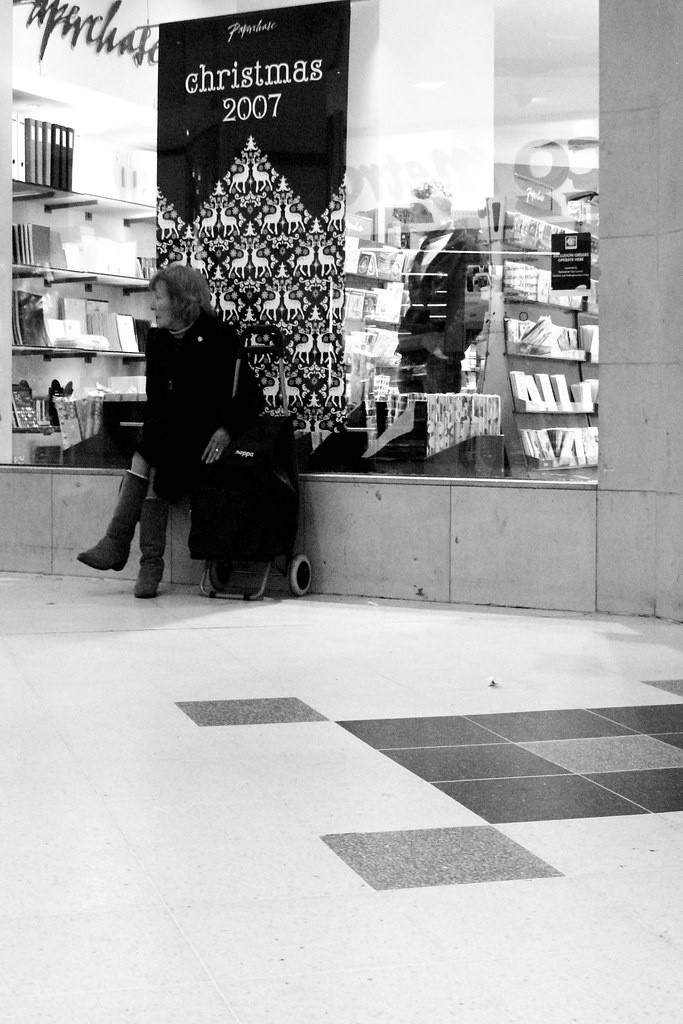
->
[345,235,407,281]
[504,316,599,363]
[509,370,598,412]
[520,427,598,468]
[10,385,50,428]
[504,210,599,261]
[343,328,398,366]
[346,283,404,322]
[11,223,51,266]
[504,260,599,314]
[13,289,157,353]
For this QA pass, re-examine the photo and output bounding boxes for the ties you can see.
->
[408,239,430,297]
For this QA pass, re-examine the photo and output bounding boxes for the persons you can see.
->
[77,266,266,599]
[395,178,490,395]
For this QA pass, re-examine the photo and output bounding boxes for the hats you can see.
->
[411,193,451,229]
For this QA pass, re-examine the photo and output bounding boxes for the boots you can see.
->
[134,496,168,598]
[76,470,149,571]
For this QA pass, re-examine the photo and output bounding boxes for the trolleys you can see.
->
[200,326,312,601]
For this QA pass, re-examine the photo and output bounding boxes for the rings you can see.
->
[215,448,219,452]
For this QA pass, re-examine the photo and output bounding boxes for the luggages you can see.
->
[187,325,312,599]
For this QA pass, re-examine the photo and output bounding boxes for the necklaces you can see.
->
[169,320,196,334]
